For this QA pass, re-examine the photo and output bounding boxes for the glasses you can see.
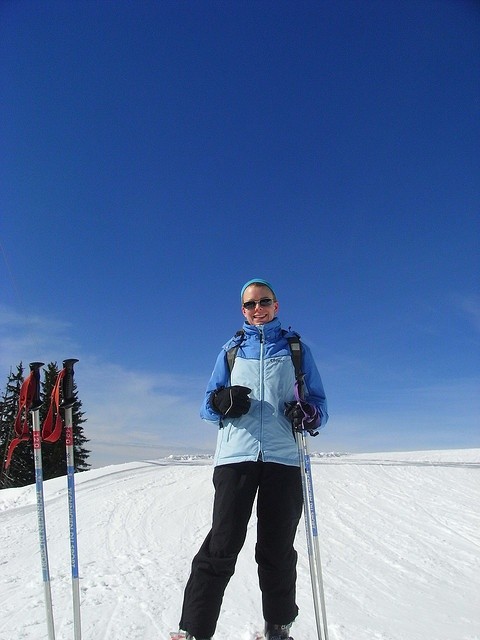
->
[242,299,274,310]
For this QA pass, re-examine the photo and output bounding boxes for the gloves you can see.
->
[285,400,320,432]
[213,385,251,418]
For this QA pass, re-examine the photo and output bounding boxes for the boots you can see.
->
[170,629,195,640]
[264,622,292,639]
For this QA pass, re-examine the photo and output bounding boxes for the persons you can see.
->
[169,278,329,640]
